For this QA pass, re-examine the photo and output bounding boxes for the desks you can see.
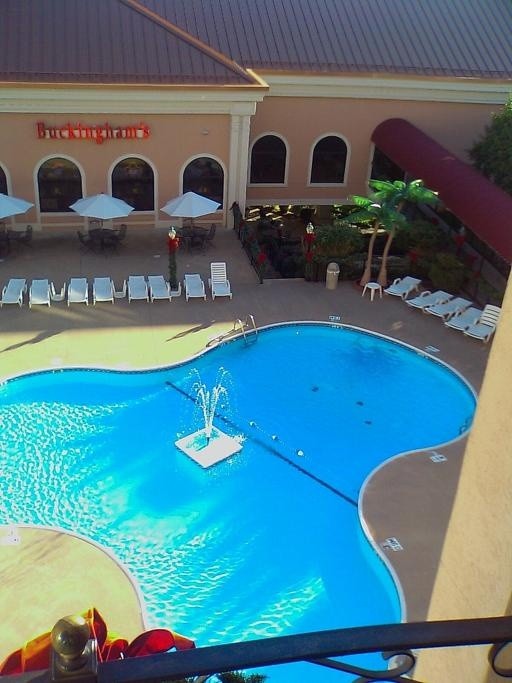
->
[362,282,382,302]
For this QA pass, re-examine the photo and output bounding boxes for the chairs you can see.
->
[169,218,216,256]
[77,220,127,260]
[0,262,233,308]
[0,224,33,257]
[383,276,502,345]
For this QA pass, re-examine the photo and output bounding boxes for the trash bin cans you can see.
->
[326,263,340,290]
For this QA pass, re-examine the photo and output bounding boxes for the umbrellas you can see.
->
[69,191,136,229]
[159,190,223,230]
[1,191,35,234]
